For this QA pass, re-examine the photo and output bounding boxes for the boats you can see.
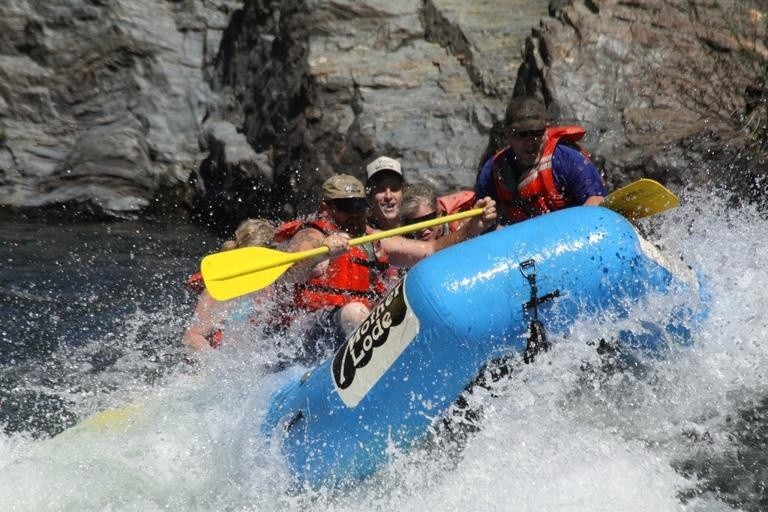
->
[50,211,721,484]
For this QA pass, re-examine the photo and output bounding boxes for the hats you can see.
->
[504,97,546,132]
[365,156,404,184]
[321,174,367,201]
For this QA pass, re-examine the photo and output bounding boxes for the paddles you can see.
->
[597,179,680,222]
[82,401,138,429]
[200,206,486,304]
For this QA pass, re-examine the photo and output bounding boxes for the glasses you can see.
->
[405,212,437,225]
[509,128,546,139]
[329,199,365,212]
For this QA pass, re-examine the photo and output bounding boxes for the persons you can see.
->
[179,217,295,385]
[473,93,608,233]
[361,154,415,240]
[277,172,498,360]
[397,185,504,243]
